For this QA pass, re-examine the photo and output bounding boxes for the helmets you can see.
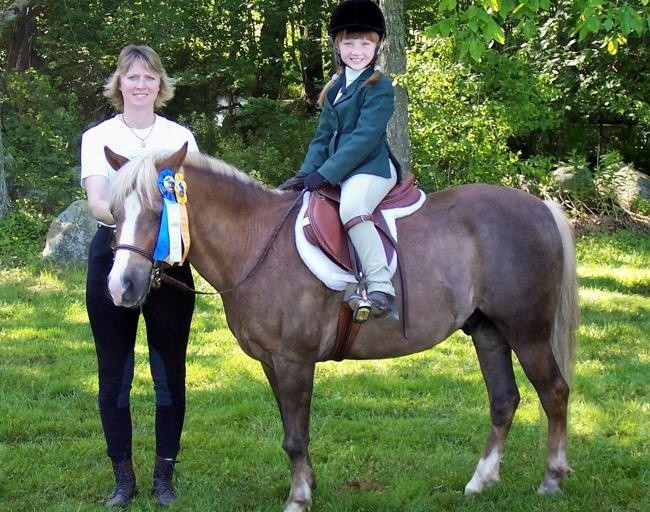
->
[329,0,386,38]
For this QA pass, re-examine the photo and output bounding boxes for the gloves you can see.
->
[278,172,325,191]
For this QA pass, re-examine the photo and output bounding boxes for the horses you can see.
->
[102,144,579,512]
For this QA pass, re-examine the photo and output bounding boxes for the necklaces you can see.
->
[122,113,155,147]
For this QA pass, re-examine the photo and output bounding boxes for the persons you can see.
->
[80,44,199,508]
[291,1,402,321]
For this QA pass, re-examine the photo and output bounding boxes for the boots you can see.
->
[104,456,139,509]
[151,456,178,508]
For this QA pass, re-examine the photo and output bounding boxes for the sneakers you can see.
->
[368,290,392,309]
